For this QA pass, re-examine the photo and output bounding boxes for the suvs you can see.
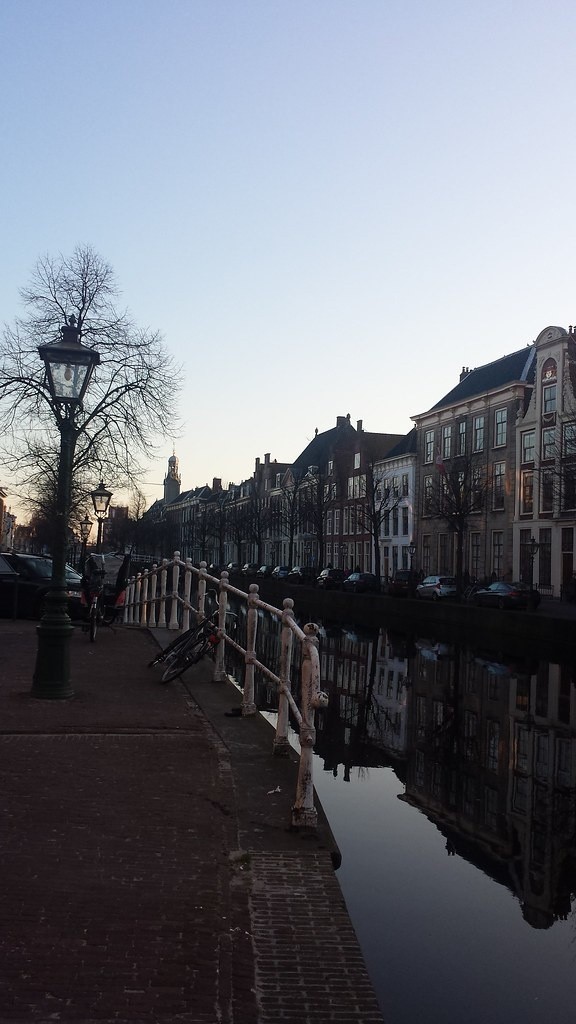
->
[317,568,347,590]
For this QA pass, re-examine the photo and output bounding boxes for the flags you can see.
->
[435,446,447,475]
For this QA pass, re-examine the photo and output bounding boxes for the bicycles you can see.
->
[148,589,240,685]
[86,552,121,642]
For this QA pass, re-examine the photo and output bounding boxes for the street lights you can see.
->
[305,546,311,566]
[90,479,113,555]
[79,513,93,575]
[407,542,417,570]
[73,533,80,565]
[527,536,540,612]
[69,539,75,566]
[32,314,101,698]
[340,544,348,572]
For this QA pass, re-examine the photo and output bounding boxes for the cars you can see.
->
[414,575,457,601]
[339,573,376,593]
[392,570,418,596]
[471,581,542,611]
[256,566,274,580]
[0,552,84,620]
[287,566,316,584]
[242,563,259,577]
[271,566,289,582]
